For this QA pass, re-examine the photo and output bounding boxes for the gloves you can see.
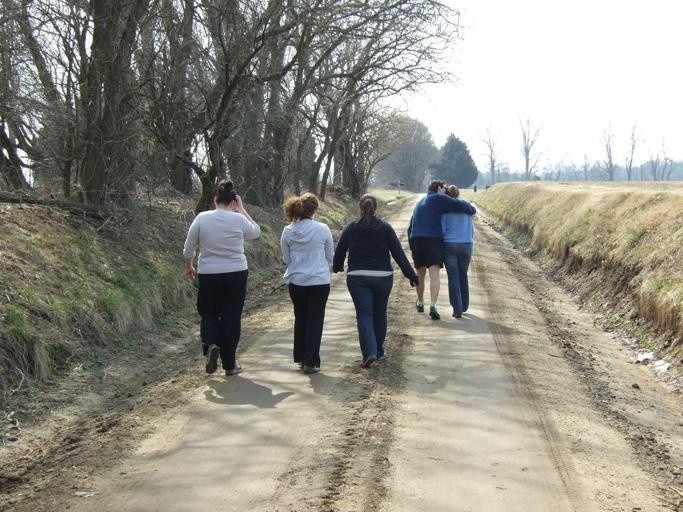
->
[410,276,419,287]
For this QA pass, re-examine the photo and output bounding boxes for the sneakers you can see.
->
[304,365,320,373]
[453,314,462,318]
[360,354,375,368]
[224,360,243,374]
[205,344,219,373]
[416,300,424,312]
[377,352,388,361]
[429,305,440,319]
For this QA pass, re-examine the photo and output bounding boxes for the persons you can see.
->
[407,180,476,320]
[335,193,420,367]
[183,179,262,376]
[281,192,335,373]
[439,185,474,318]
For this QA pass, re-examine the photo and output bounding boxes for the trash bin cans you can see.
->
[474,186,476,192]
